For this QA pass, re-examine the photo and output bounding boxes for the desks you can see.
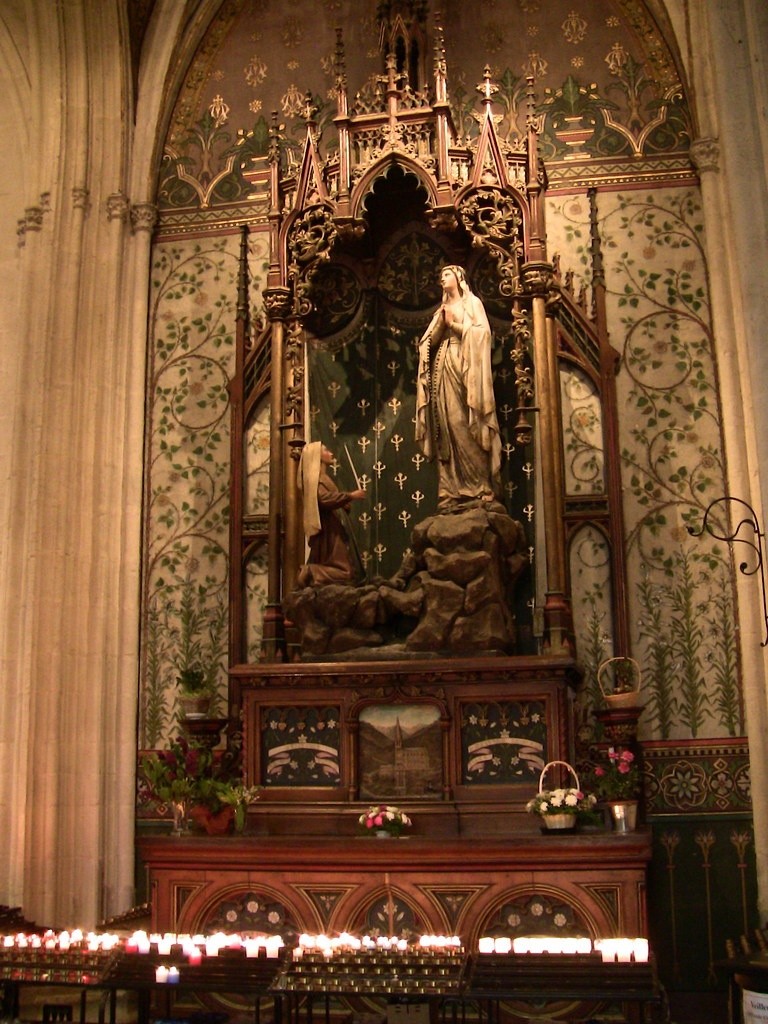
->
[131,832,659,1024]
[710,951,768,1024]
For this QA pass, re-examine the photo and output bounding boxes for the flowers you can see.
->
[196,773,262,831]
[142,736,214,801]
[527,786,598,816]
[585,745,643,800]
[357,804,412,836]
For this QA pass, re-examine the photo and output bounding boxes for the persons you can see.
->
[296,441,370,587]
[415,265,502,510]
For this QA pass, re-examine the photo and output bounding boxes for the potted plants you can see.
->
[597,655,642,707]
[174,583,230,712]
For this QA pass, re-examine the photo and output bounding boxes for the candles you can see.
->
[419,934,462,946]
[32,937,42,949]
[477,937,592,954]
[293,947,303,957]
[29,934,39,941]
[60,938,70,949]
[86,930,285,965]
[323,946,334,958]
[169,966,180,983]
[18,937,28,948]
[15,933,27,942]
[72,929,83,942]
[42,929,59,944]
[58,930,70,941]
[3,936,15,947]
[299,932,409,951]
[46,939,56,949]
[155,966,169,983]
[594,937,650,963]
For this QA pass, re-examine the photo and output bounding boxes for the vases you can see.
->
[607,799,638,832]
[190,805,234,837]
[539,761,580,829]
[171,793,191,831]
[374,829,397,839]
